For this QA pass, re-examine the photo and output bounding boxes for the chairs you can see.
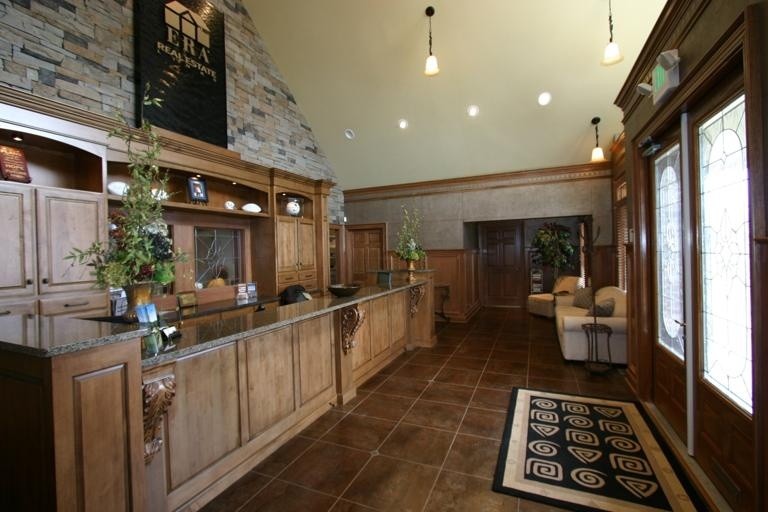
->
[527,275,585,319]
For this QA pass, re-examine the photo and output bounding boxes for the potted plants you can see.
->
[393,203,428,281]
[61,80,206,324]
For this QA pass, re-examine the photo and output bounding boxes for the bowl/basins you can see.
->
[325,283,362,298]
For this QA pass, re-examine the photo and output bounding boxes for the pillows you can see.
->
[572,286,615,317]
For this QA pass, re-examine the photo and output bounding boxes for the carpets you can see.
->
[491,385,703,512]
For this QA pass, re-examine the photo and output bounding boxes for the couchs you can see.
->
[553,283,629,363]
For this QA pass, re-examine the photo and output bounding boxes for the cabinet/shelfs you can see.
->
[0,124,112,318]
[249,187,318,299]
